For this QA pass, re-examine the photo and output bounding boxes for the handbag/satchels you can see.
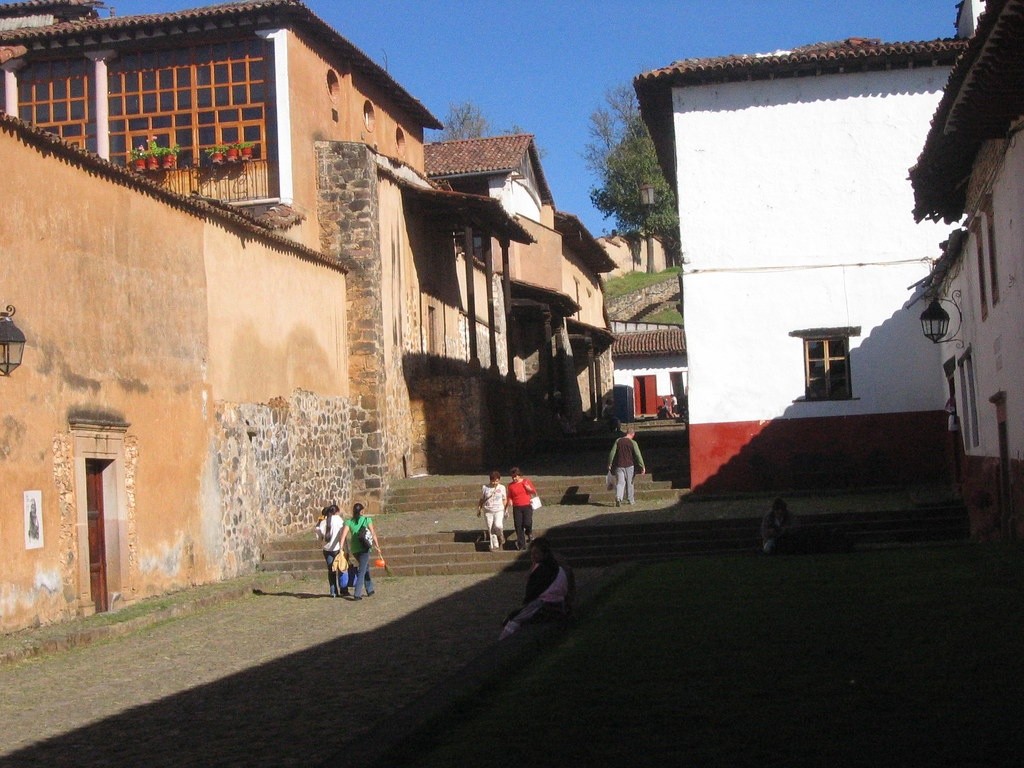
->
[358,516,373,548]
[606,469,616,491]
[331,549,360,589]
[374,548,386,568]
[526,479,542,510]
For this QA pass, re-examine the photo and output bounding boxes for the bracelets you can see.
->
[377,546,380,548]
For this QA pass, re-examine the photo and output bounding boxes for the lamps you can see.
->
[920,290,963,349]
[0,304,26,375]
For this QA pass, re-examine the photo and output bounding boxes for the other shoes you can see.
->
[616,498,620,507]
[354,596,362,601]
[368,590,375,596]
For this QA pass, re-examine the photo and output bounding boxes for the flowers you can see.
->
[204,141,251,158]
[129,145,147,160]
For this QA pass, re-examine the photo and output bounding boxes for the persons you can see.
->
[319,505,353,599]
[318,508,329,526]
[607,428,646,507]
[668,394,678,417]
[656,405,662,418]
[476,471,509,551]
[339,502,380,599]
[507,467,536,550]
[602,400,622,432]
[502,537,575,628]
[760,498,793,555]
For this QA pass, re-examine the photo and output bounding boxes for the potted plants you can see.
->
[147,136,161,156]
[161,144,179,168]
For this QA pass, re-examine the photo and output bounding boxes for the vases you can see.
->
[242,147,253,157]
[226,149,238,159]
[147,156,159,169]
[135,159,146,171]
[212,153,223,160]
